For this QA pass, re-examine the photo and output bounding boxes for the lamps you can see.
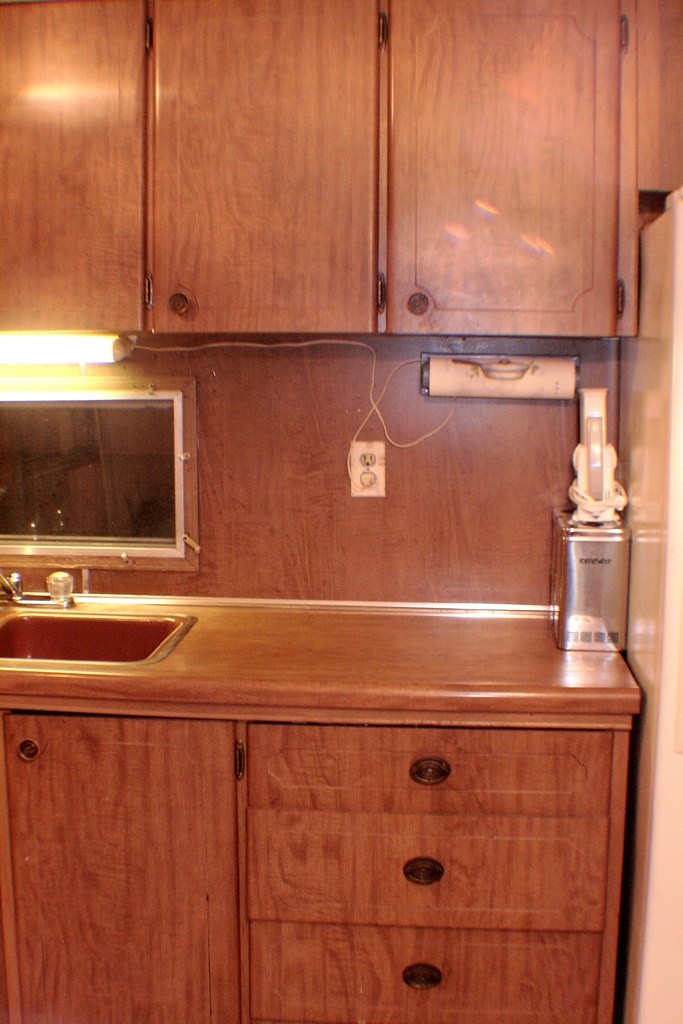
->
[0,331,460,491]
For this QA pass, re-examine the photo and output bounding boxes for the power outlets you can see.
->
[350,441,385,497]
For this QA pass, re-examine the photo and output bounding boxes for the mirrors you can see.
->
[0,376,201,576]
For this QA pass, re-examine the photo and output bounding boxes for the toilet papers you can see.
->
[427,350,580,403]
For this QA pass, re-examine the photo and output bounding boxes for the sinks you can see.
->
[0,610,198,664]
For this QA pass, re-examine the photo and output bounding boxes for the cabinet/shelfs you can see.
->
[237,720,632,1023]
[2,708,244,1024]
[0,0,683,338]
